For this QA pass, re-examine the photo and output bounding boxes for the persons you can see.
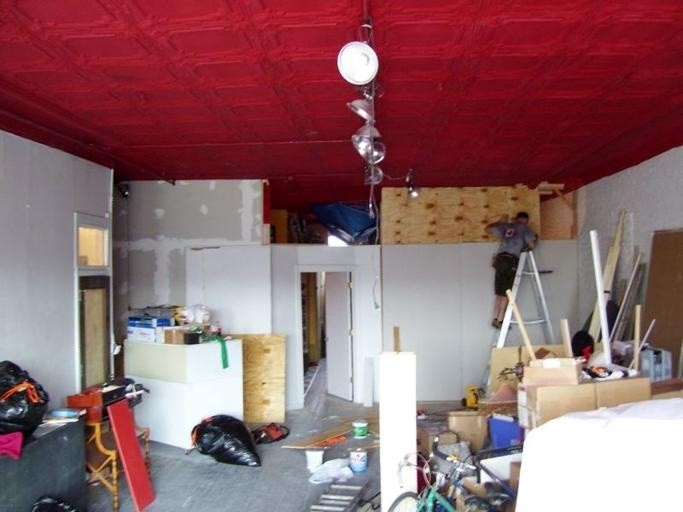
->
[485,211,539,328]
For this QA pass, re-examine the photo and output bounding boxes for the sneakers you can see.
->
[493,318,512,330]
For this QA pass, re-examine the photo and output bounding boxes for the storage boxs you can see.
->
[421,357,683,468]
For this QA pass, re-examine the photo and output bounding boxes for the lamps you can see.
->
[336,19,419,199]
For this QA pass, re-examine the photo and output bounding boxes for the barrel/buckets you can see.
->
[352,416,369,440]
[349,448,368,472]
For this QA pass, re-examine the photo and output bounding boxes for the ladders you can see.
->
[481,247,558,391]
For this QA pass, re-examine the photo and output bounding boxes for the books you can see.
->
[287,212,310,244]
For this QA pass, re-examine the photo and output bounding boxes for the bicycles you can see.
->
[387,455,492,512]
[422,434,513,511]
